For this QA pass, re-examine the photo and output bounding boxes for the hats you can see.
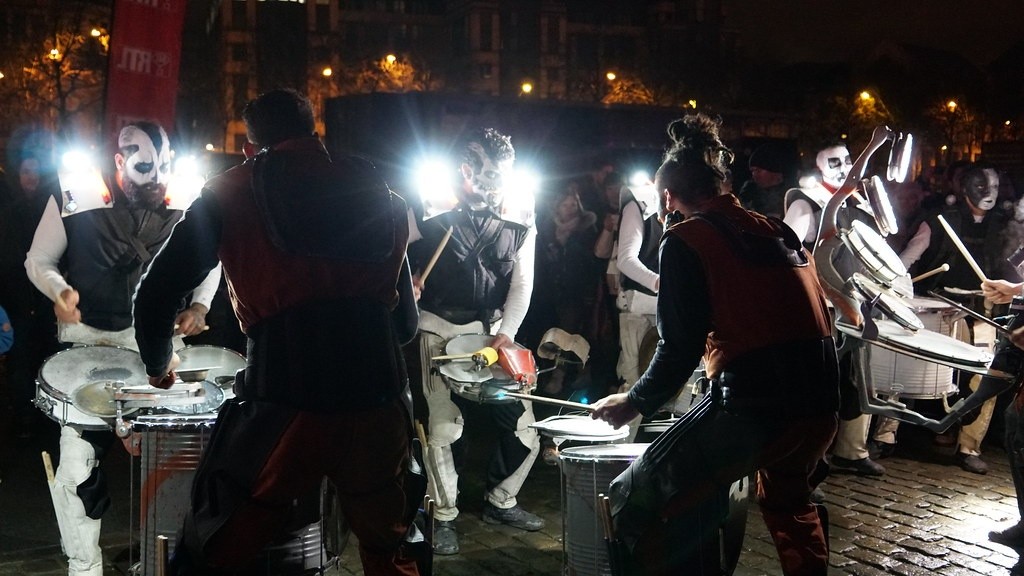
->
[747,147,779,173]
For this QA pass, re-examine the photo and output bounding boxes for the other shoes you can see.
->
[987,519,1024,543]
[483,499,545,530]
[810,486,826,503]
[433,516,458,553]
[874,436,897,456]
[832,450,884,477]
[954,449,990,474]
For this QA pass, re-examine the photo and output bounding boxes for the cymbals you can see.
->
[172,368,210,383]
[72,378,140,418]
[439,361,494,383]
[163,379,226,413]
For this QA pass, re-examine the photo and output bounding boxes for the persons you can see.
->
[590,115,841,576]
[1,124,224,575]
[407,130,664,553]
[744,125,1024,547]
[132,92,432,576]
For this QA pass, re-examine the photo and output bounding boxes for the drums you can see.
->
[132,414,331,576]
[437,332,539,405]
[34,343,149,431]
[835,314,996,367]
[540,414,630,442]
[862,174,899,238]
[846,271,925,333]
[886,130,913,184]
[172,344,248,399]
[559,441,652,576]
[845,220,905,287]
[851,296,963,400]
